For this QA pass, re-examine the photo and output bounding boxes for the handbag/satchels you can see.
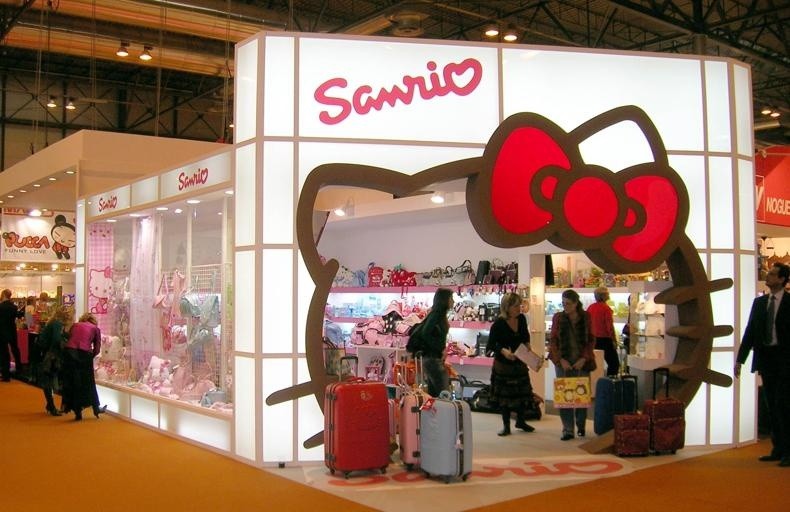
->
[471,388,497,413]
[326,323,346,344]
[583,358,597,372]
[202,389,226,407]
[324,346,351,377]
[351,315,412,349]
[524,397,542,421]
[478,302,500,322]
[368,260,518,287]
[405,322,424,351]
[151,290,220,351]
[553,376,592,409]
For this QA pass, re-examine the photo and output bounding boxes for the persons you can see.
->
[25,296,37,328]
[61,312,108,419]
[0,289,24,382]
[545,290,597,440]
[485,293,544,436]
[587,286,621,378]
[421,288,455,398]
[32,304,76,417]
[734,263,789,467]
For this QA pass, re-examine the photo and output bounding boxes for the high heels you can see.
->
[94,405,108,419]
[46,403,63,416]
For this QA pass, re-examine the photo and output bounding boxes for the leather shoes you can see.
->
[759,455,780,461]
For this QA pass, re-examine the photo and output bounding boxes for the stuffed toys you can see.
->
[91,334,126,381]
[330,266,347,288]
[379,269,390,287]
[337,271,356,288]
[152,367,169,390]
[142,356,171,384]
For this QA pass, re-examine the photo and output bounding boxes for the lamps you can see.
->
[430,190,446,204]
[115,38,154,61]
[45,94,79,110]
[484,14,520,42]
[760,103,782,118]
[334,195,354,216]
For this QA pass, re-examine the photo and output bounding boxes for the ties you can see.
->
[765,296,776,345]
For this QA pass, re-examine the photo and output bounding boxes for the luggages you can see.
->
[323,355,390,480]
[399,351,473,483]
[594,368,686,458]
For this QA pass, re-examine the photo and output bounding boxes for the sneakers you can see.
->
[515,421,535,432]
[497,429,510,436]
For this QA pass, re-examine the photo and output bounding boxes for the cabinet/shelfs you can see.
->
[322,281,516,369]
[543,280,666,365]
